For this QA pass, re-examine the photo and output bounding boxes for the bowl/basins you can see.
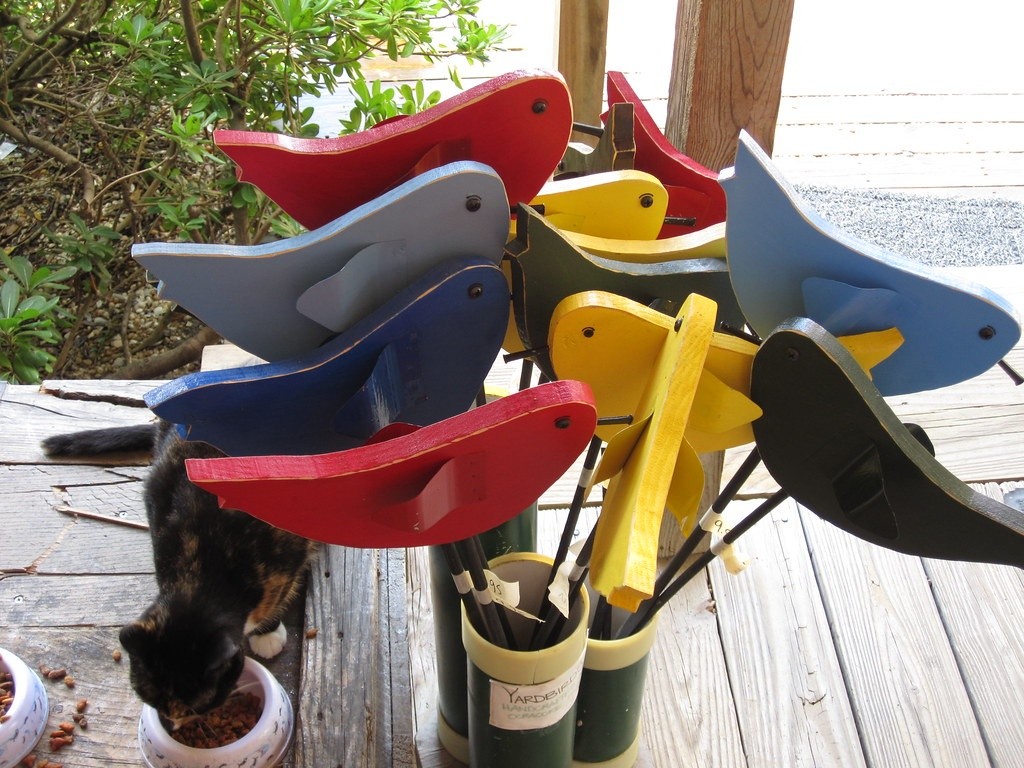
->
[0,647,49,767]
[137,656,295,768]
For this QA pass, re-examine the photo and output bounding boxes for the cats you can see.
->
[40,418,318,732]
